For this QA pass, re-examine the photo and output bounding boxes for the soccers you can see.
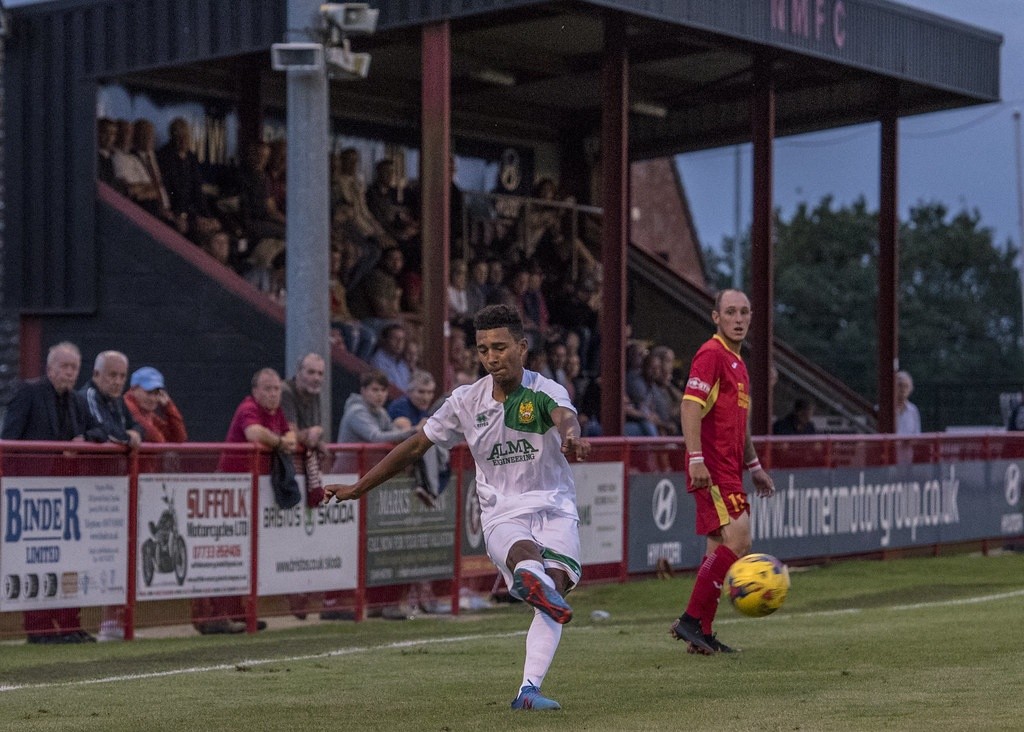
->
[726,552,791,619]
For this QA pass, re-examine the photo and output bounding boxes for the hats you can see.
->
[269,447,302,509]
[130,367,165,393]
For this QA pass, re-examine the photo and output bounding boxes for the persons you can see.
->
[323,305,591,712]
[97,115,696,635]
[664,288,775,656]
[873,370,921,435]
[0,341,188,645]
[771,397,816,435]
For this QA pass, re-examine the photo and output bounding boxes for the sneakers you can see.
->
[513,568,573,624]
[687,635,733,655]
[511,679,561,711]
[670,618,714,656]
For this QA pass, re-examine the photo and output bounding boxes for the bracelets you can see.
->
[277,437,285,449]
[688,451,704,461]
[746,458,760,469]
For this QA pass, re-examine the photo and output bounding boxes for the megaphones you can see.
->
[320,2,380,34]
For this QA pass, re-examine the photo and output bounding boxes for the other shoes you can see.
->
[200,619,246,635]
[25,632,76,643]
[61,629,96,641]
[282,583,488,620]
[98,630,146,643]
